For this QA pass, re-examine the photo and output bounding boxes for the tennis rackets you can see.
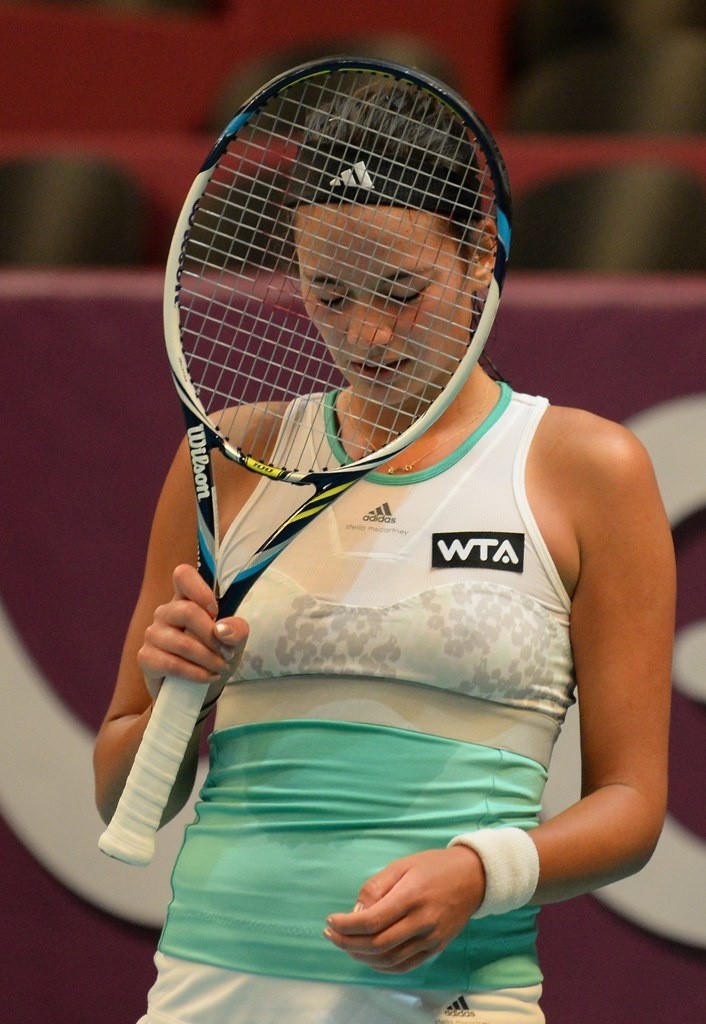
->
[99,54,510,866]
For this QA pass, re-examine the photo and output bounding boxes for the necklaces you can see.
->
[347,383,489,475]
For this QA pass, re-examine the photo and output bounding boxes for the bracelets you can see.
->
[445,826,540,920]
[150,686,226,727]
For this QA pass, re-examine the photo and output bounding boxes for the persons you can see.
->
[91,70,678,1024]
